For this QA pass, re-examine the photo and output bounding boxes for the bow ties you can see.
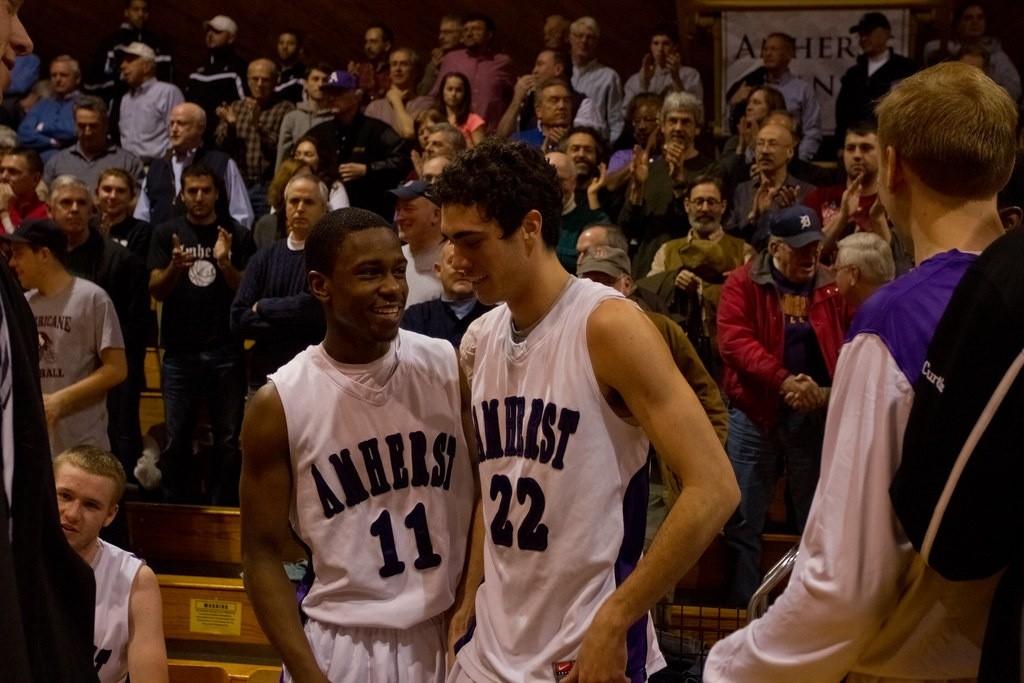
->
[166,149,187,162]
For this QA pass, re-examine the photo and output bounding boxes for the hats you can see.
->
[113,42,155,62]
[849,12,891,33]
[388,180,443,209]
[576,245,631,279]
[204,15,237,37]
[768,206,827,248]
[318,72,355,91]
[0,219,67,252]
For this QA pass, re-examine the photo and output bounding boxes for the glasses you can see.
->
[827,263,857,278]
[687,198,723,210]
[753,141,793,152]
[321,88,348,97]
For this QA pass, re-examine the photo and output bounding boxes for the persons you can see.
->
[427,133,742,683]
[0,0,1024,683]
